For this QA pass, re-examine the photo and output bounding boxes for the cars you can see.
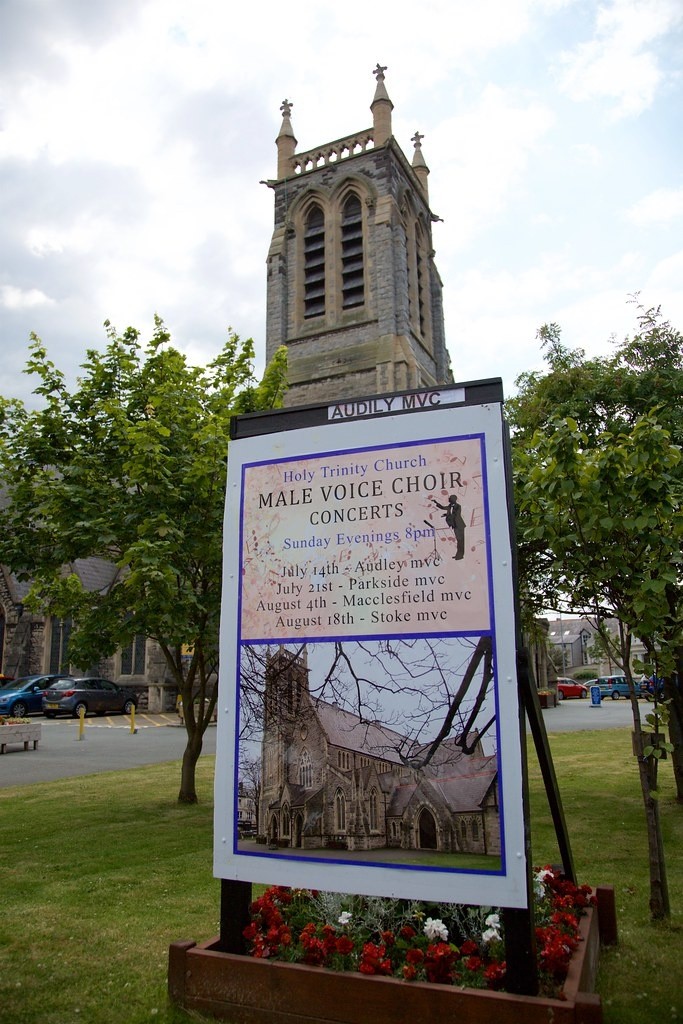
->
[0,674,16,689]
[40,675,139,719]
[597,674,641,700]
[0,673,77,718]
[582,679,598,692]
[639,674,679,702]
[557,676,588,700]
